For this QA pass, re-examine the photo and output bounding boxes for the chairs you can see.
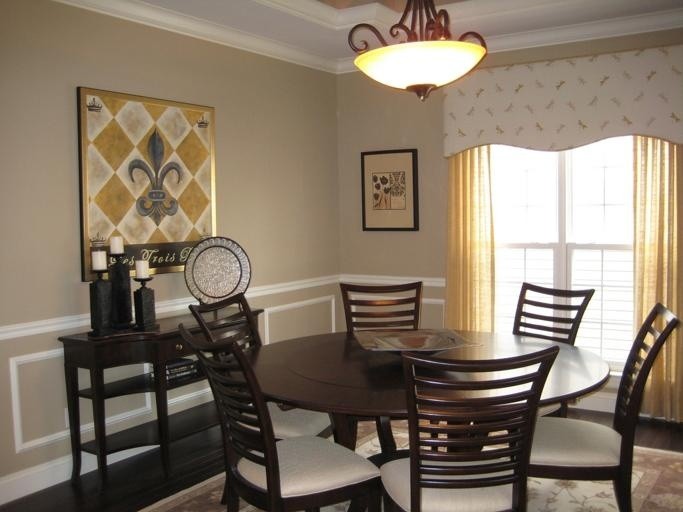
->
[188,292,334,505]
[178,322,381,512]
[528,302,679,511]
[339,281,424,453]
[512,282,595,418]
[380,344,560,512]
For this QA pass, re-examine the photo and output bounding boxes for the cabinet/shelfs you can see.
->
[57,305,264,486]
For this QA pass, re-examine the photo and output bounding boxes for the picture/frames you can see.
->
[360,148,419,232]
[76,85,217,283]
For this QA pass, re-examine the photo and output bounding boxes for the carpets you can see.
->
[134,421,683,512]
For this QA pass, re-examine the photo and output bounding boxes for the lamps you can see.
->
[347,0,489,103]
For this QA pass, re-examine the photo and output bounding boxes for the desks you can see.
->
[228,328,611,512]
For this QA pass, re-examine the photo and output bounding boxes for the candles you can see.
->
[133,260,153,282]
[109,236,125,257]
[90,251,108,273]
